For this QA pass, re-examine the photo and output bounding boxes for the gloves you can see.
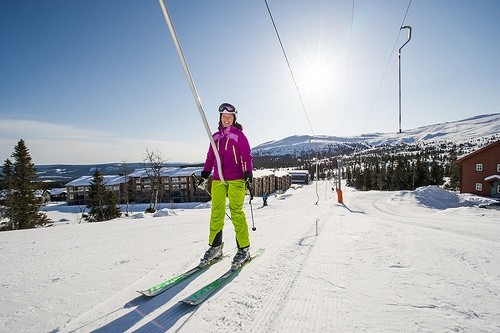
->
[244,171,253,191]
[197,171,211,189]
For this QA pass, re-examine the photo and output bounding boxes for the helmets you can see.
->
[219,103,238,119]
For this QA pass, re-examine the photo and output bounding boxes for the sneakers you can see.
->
[204,245,220,258]
[234,248,250,262]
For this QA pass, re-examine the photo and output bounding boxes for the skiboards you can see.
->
[257,204,268,209]
[136,248,265,306]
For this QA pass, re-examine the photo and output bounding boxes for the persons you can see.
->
[262,192,268,206]
[198,103,252,263]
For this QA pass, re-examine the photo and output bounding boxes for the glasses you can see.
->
[219,103,235,112]
